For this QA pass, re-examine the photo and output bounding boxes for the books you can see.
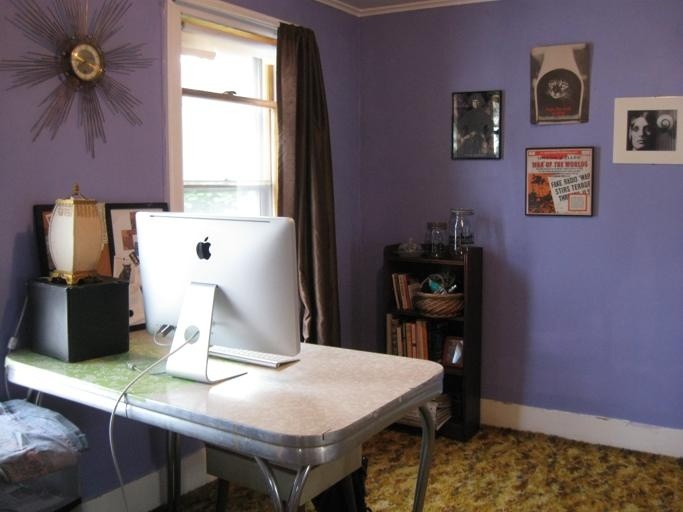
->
[396,393,454,431]
[385,313,463,368]
[392,272,419,311]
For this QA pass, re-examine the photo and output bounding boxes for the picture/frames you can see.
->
[525,146,595,217]
[451,90,503,160]
[33,204,56,278]
[105,202,170,332]
[612,95,683,165]
[530,41,593,126]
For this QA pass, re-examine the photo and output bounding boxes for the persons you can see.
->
[454,94,493,158]
[628,112,658,151]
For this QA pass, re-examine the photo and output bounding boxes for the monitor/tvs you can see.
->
[134,211,302,385]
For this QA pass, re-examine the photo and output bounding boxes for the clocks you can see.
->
[60,32,106,91]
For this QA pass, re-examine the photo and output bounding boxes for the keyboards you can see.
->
[205,344,304,369]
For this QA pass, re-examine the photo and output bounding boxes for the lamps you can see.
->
[48,183,104,285]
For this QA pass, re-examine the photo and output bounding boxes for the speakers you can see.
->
[29,275,130,364]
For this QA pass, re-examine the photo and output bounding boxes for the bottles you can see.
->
[447,207,474,261]
[425,220,447,260]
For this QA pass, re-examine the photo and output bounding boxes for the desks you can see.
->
[4,328,445,512]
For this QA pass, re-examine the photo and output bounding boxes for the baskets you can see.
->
[414,274,464,318]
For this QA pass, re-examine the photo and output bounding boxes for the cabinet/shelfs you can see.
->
[383,242,483,443]
